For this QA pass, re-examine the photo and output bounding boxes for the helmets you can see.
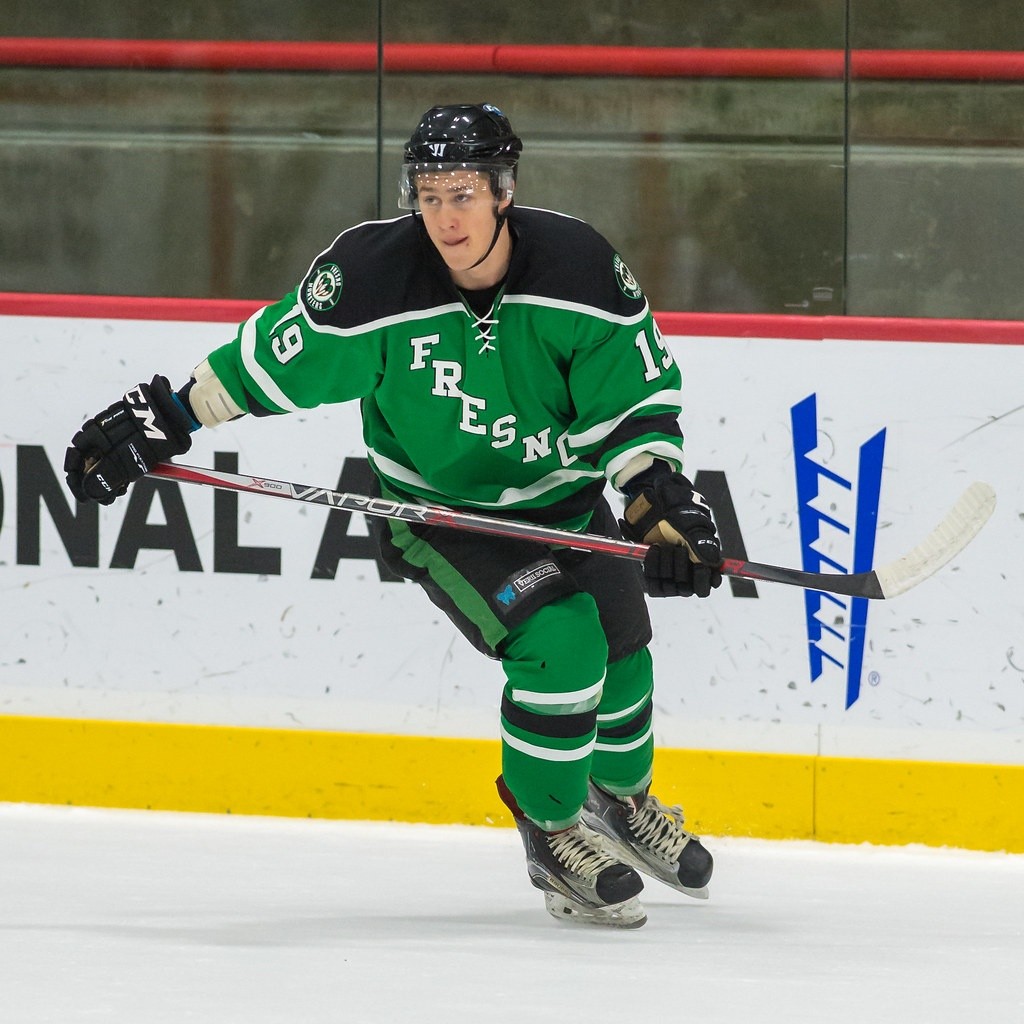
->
[404,102,523,200]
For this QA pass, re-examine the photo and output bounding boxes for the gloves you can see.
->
[64,375,192,506]
[618,473,724,598]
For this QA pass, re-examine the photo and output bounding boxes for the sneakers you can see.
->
[583,775,714,900]
[496,774,649,929]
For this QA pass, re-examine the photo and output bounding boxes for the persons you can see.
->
[63,103,721,927]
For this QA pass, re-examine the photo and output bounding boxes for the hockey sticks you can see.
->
[144,460,999,602]
